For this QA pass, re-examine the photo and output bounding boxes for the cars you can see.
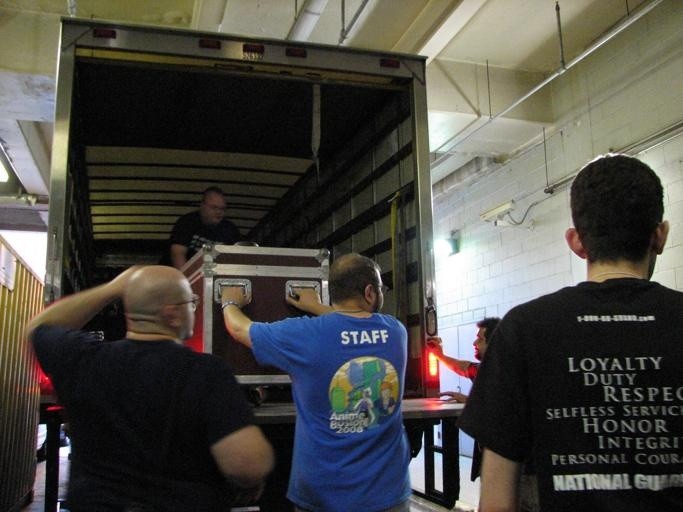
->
[37,423,70,461]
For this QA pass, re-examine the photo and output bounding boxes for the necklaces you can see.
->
[586,270,643,280]
[332,307,366,314]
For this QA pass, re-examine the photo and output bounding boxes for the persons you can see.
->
[426,316,503,404]
[449,152,683,512]
[24,261,278,511]
[168,186,249,269]
[220,252,413,512]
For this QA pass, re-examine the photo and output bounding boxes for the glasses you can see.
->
[176,294,200,308]
[368,282,388,295]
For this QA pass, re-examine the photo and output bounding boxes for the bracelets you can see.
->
[222,299,241,309]
